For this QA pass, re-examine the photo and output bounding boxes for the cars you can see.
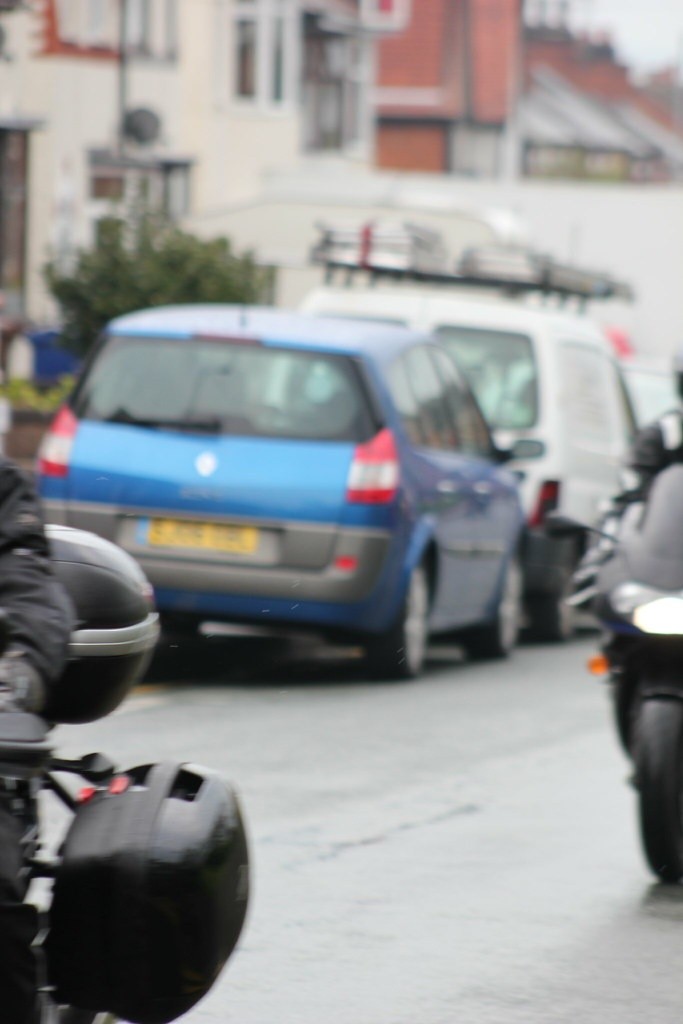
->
[615,355,682,434]
[310,281,683,646]
[33,300,526,678]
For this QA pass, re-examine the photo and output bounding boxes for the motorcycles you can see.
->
[0,525,251,1023]
[572,350,683,884]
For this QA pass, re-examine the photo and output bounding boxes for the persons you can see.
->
[574,360,682,787]
[0,459,72,1024]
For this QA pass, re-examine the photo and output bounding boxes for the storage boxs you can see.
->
[60,760,249,1024]
[43,522,160,725]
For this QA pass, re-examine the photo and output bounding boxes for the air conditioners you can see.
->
[331,0,411,32]
